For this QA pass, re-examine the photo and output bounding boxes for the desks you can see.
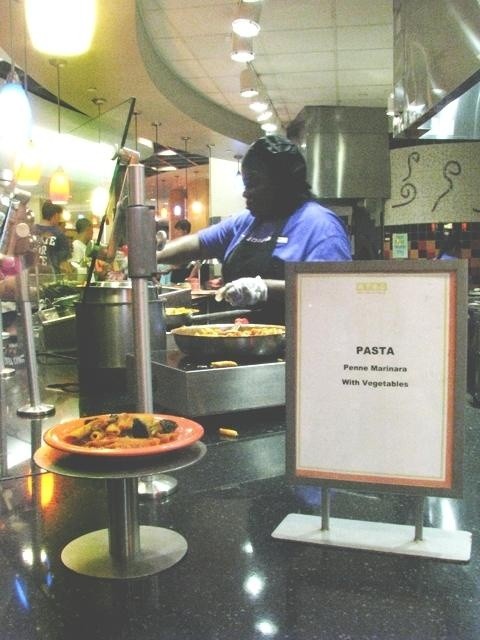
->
[2,400,478,639]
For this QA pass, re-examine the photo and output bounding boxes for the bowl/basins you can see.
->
[76,280,160,305]
[170,324,287,362]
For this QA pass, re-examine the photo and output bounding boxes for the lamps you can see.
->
[232,1,280,133]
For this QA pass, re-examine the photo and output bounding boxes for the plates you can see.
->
[43,412,205,459]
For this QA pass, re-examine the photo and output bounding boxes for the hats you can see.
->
[241,135,306,181]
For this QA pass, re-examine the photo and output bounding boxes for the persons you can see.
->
[433,236,462,261]
[156,134,353,326]
[0,199,219,301]
